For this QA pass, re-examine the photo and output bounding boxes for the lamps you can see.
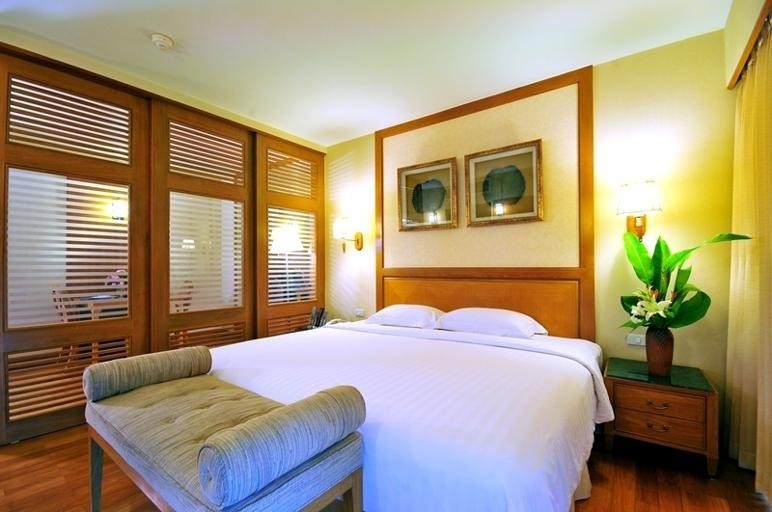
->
[334,216,365,251]
[271,228,304,302]
[619,180,660,241]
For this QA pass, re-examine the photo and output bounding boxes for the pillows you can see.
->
[196,383,366,509]
[81,345,212,402]
[365,303,442,327]
[434,305,549,339]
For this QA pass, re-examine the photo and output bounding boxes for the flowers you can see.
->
[616,230,751,340]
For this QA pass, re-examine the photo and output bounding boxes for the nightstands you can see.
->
[601,356,722,480]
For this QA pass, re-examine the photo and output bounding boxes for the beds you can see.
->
[206,267,614,512]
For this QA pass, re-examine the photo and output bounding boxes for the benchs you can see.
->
[79,345,368,512]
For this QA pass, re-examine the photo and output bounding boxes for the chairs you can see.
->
[51,282,195,372]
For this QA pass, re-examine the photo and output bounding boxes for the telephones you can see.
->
[315,308,324,327]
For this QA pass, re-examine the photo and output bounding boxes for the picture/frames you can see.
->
[397,154,459,233]
[464,139,544,227]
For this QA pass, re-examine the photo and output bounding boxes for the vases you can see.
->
[645,324,674,377]
